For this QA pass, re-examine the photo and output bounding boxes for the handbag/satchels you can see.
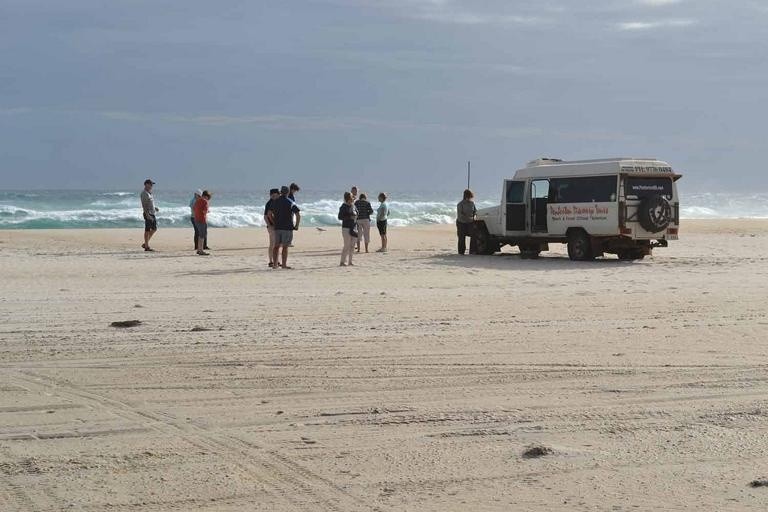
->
[350,221,360,238]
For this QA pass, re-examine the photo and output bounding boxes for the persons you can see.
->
[375,192,391,252]
[140,179,159,252]
[338,185,373,267]
[264,182,300,269]
[189,189,209,250]
[456,190,478,255]
[193,191,212,256]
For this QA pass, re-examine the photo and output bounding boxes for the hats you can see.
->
[194,188,202,197]
[270,186,289,194]
[144,179,156,184]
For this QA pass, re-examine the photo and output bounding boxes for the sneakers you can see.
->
[141,243,155,251]
[339,245,387,266]
[268,262,291,270]
[288,244,294,247]
[192,246,212,255]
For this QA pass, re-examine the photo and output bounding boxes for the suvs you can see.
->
[469,157,682,262]
